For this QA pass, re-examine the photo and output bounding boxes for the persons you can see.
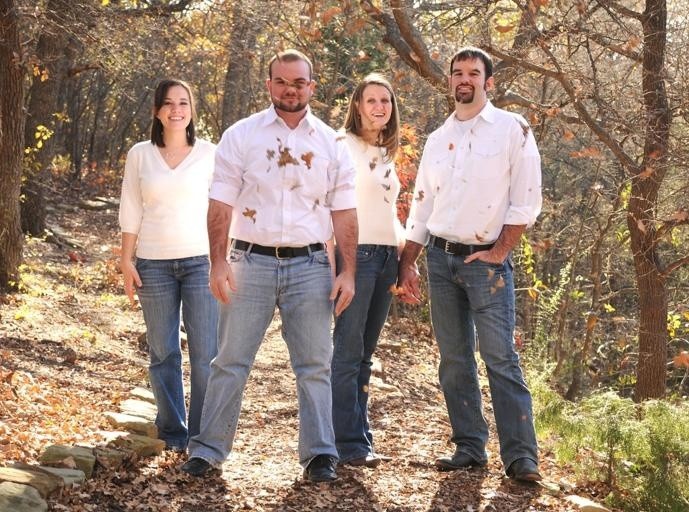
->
[181,49,359,483]
[333,73,421,468]
[397,47,543,480]
[118,80,219,454]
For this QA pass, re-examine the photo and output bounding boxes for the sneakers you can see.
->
[305,454,338,482]
[507,458,542,481]
[435,451,478,471]
[179,457,217,477]
[349,455,381,468]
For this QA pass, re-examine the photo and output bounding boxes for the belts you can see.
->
[430,234,493,256]
[231,238,324,260]
